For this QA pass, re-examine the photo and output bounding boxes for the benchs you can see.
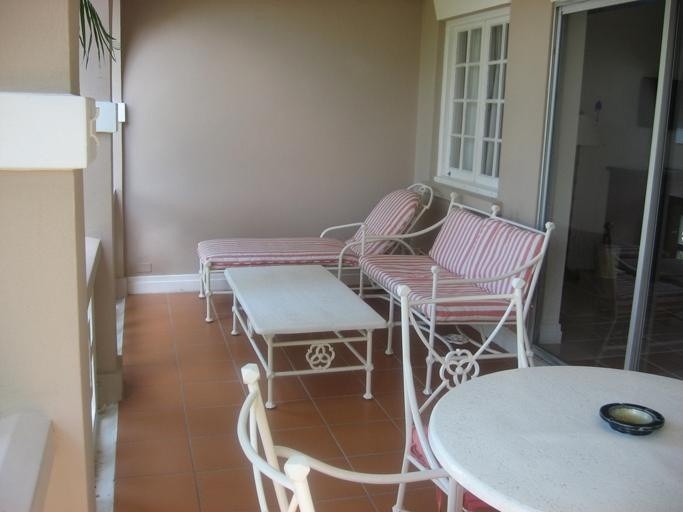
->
[358,194,556,397]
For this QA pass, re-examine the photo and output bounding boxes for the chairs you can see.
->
[392,278,538,510]
[199,182,434,323]
[235,362,463,511]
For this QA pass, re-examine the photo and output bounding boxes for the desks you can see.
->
[426,367,682,512]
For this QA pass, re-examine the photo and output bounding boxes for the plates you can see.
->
[600,402,664,436]
